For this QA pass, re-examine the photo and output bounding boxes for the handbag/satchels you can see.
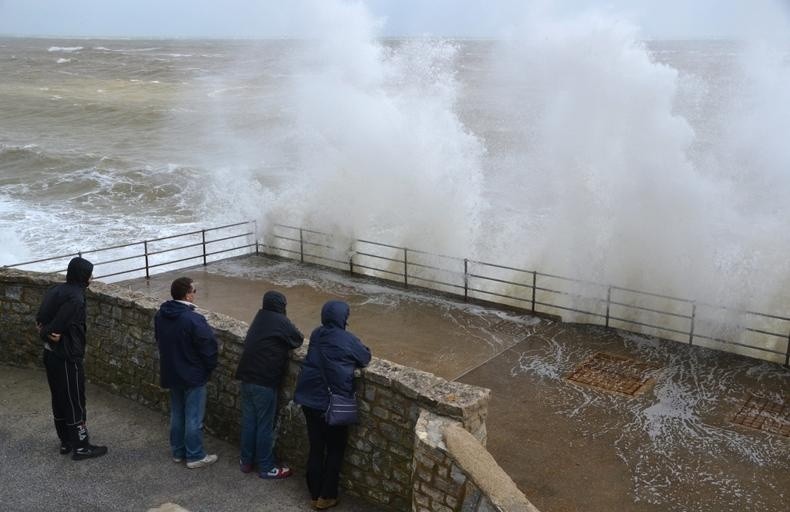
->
[322,391,361,428]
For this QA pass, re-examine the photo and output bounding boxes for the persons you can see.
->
[293,300,373,511]
[234,288,305,480]
[153,277,218,470]
[35,257,109,460]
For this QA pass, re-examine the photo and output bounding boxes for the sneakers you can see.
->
[258,464,292,479]
[185,452,218,470]
[310,495,342,510]
[59,443,74,454]
[72,445,107,461]
[172,457,183,462]
[239,458,254,473]
